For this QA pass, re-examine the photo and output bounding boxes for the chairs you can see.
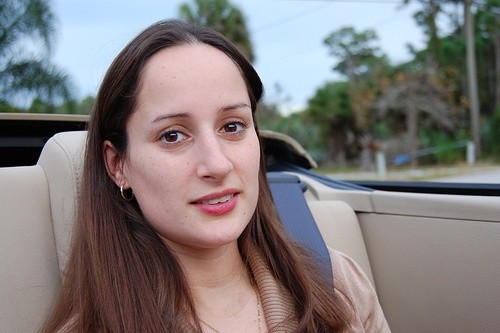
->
[0,131,376,333]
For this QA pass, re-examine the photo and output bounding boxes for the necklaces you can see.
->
[181,265,261,332]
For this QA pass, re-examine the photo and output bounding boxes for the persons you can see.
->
[32,18,393,333]
